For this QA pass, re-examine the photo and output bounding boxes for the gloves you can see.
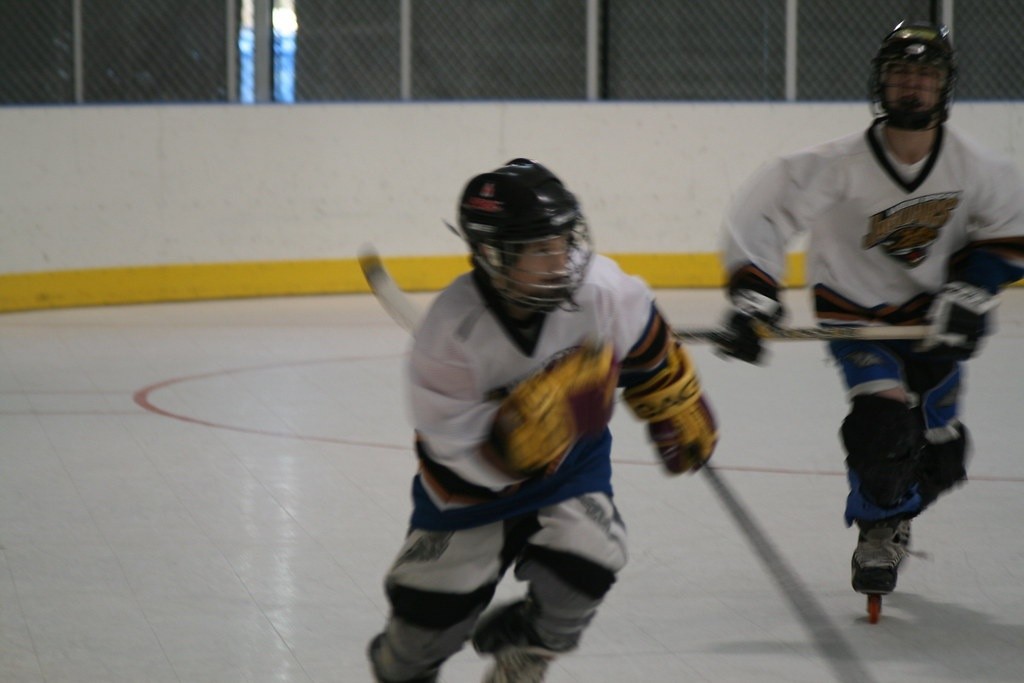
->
[717,263,783,366]
[912,246,1024,359]
[487,335,619,476]
[620,339,719,476]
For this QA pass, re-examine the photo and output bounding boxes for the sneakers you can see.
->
[851,516,912,625]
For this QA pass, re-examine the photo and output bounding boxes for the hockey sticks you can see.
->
[358,243,941,343]
[693,443,876,683]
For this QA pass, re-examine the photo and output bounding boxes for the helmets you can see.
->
[869,20,955,132]
[459,158,596,314]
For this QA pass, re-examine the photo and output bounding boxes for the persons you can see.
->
[715,20,1024,595]
[367,160,719,683]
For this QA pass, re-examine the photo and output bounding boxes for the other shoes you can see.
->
[369,632,438,683]
[473,603,548,683]
